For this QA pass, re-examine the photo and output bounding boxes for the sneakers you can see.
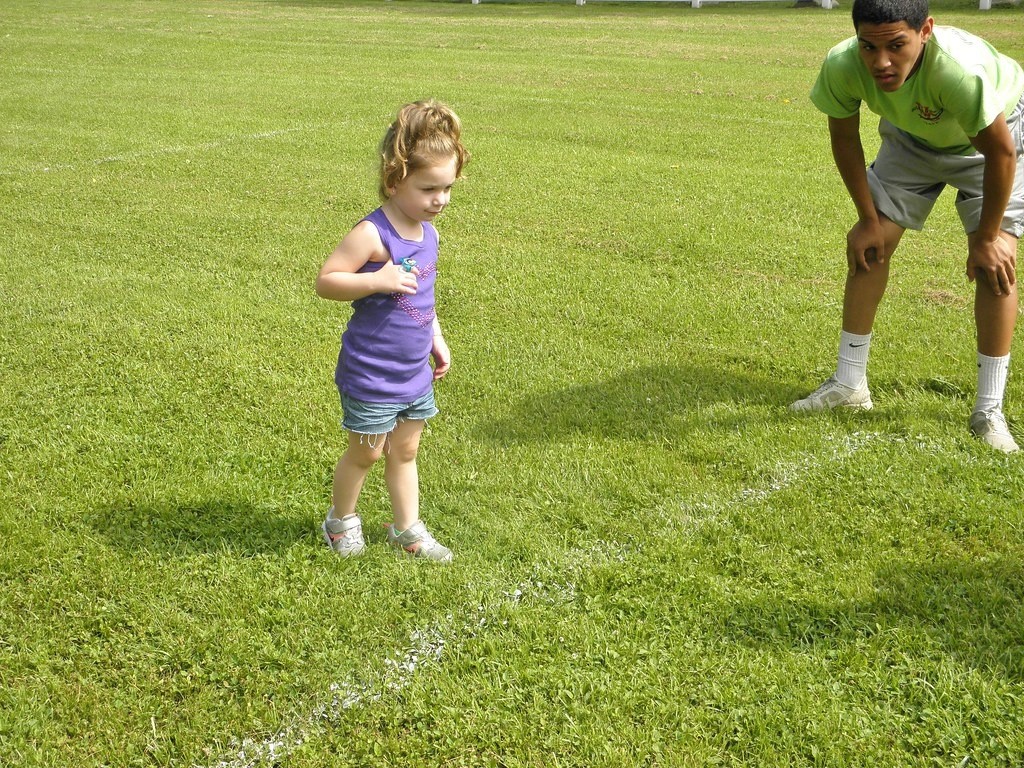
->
[789,372,873,414]
[970,402,1019,455]
[321,505,370,563]
[383,519,454,566]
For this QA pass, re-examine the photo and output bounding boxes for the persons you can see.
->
[314,102,470,561]
[789,0,1024,451]
